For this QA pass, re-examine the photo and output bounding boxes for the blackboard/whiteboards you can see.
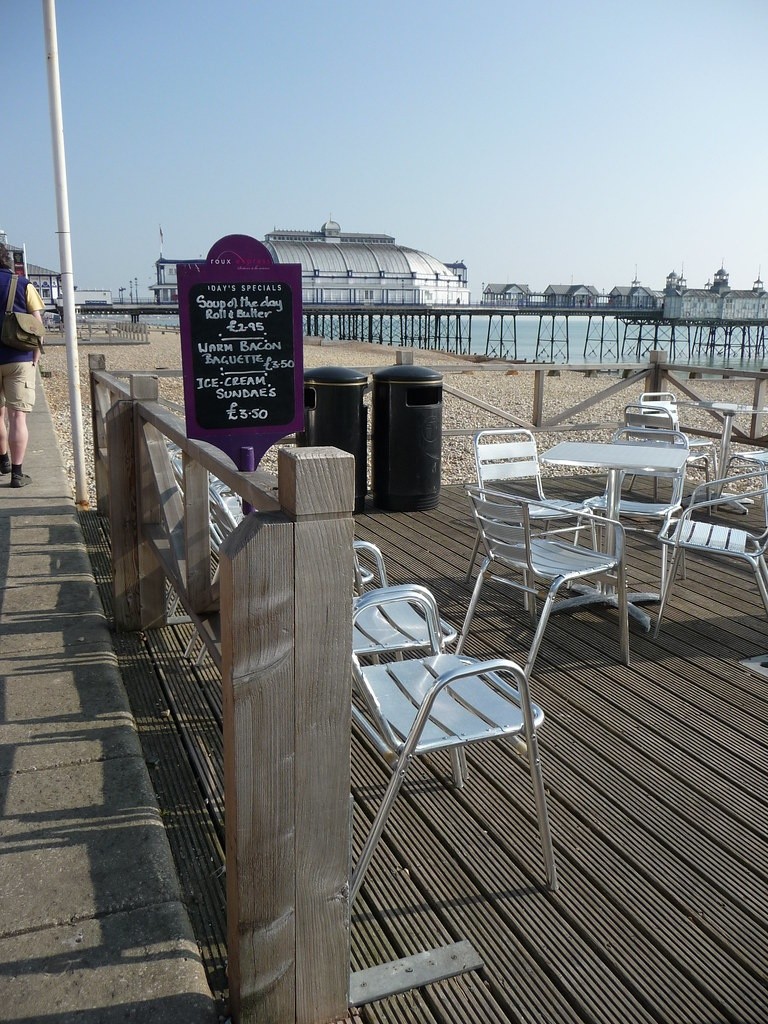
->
[175,262,305,435]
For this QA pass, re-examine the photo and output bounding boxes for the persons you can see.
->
[0,242,45,489]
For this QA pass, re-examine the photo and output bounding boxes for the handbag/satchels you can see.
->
[1,275,46,354]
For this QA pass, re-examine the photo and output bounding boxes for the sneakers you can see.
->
[0,462,11,476]
[11,474,31,487]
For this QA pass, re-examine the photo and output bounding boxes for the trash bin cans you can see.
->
[372,364,444,512]
[296,366,369,515]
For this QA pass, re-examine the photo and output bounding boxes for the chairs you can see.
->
[172,391,768,908]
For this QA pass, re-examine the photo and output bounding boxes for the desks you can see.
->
[670,400,768,516]
[539,441,689,633]
